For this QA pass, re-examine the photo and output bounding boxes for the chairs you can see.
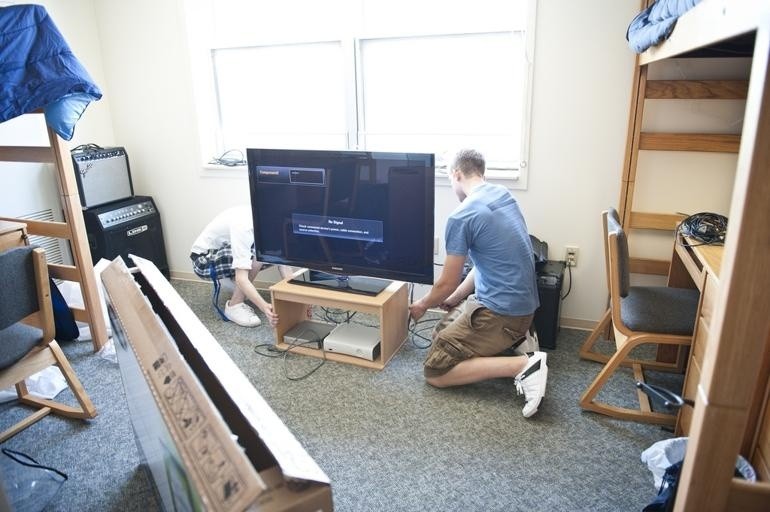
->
[1,246,97,445]
[577,206,699,428]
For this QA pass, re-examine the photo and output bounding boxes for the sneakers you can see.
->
[224,299,262,328]
[514,351,548,418]
[511,321,540,356]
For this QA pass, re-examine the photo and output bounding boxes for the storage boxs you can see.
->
[101,254,333,511]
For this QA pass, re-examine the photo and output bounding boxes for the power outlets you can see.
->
[566,247,578,266]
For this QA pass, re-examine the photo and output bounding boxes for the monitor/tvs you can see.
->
[245,147,436,297]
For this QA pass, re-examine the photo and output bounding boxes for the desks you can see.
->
[268,268,410,369]
[655,220,724,436]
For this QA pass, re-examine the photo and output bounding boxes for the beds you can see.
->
[2,3,110,353]
[619,0,768,511]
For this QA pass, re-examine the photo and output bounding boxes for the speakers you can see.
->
[83,196,170,282]
[533,260,567,350]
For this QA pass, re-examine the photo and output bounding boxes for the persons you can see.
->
[190,202,313,331]
[408,147,551,418]
